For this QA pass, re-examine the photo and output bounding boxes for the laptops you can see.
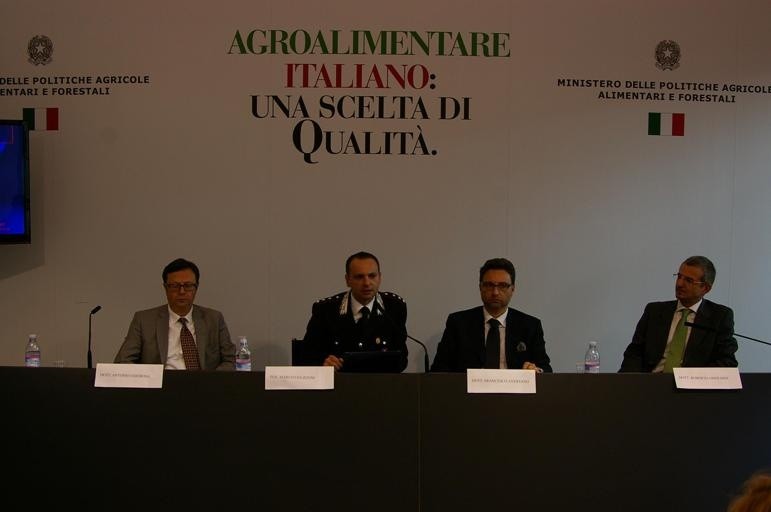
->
[344,352,400,373]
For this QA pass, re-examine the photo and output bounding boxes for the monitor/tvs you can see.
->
[0,119,31,245]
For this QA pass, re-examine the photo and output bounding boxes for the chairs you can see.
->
[290,337,304,362]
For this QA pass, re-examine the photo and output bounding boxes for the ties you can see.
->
[355,306,374,337]
[661,308,691,374]
[485,318,502,369]
[176,318,203,370]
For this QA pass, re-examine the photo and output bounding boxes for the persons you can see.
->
[428,259,554,374]
[302,251,408,373]
[113,258,238,372]
[726,468,771,512]
[616,256,740,374]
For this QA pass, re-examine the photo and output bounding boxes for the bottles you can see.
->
[237,337,252,372]
[24,332,42,367]
[585,340,601,373]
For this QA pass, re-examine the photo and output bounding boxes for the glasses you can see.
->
[672,273,706,286]
[479,282,513,291]
[165,282,198,291]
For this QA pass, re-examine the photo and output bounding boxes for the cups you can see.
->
[575,360,585,373]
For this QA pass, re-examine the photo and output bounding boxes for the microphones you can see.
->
[376,302,430,374]
[87,305,102,368]
[684,321,771,346]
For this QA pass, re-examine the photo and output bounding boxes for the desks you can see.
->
[421,370,771,512]
[1,364,422,510]
